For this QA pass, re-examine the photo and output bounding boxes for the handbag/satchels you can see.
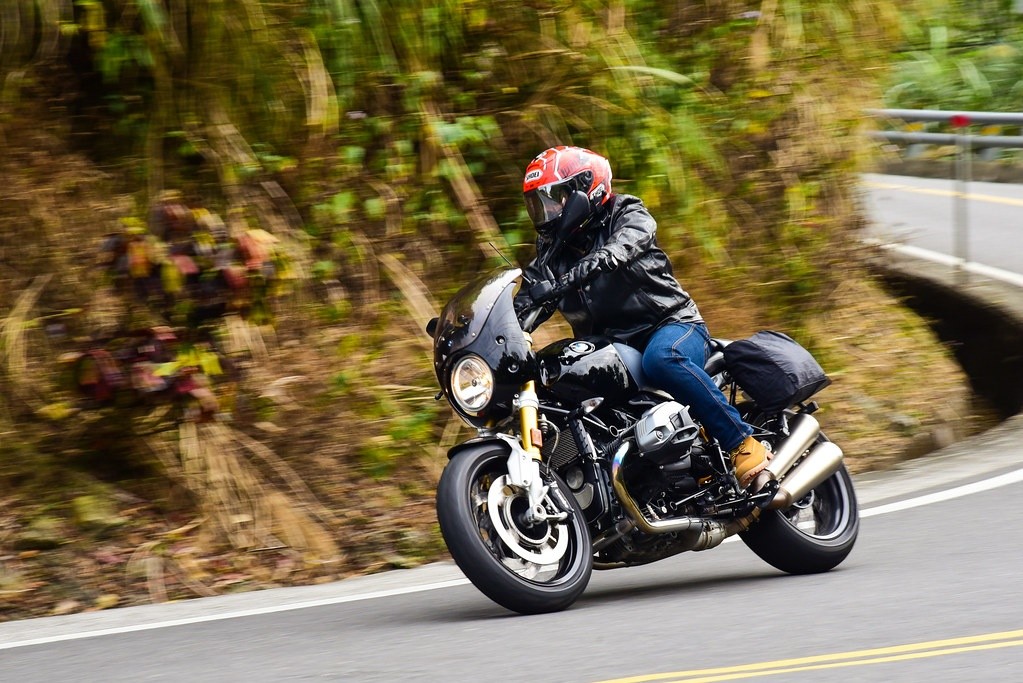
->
[723,330,825,409]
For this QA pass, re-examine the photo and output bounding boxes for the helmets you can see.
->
[524,146,613,227]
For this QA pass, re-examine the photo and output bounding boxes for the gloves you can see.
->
[547,272,572,301]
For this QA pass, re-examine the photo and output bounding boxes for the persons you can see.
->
[513,146,774,481]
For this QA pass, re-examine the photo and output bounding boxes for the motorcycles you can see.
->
[424,187,861,614]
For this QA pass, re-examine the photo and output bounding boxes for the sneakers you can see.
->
[593,554,627,570]
[729,435,774,488]
